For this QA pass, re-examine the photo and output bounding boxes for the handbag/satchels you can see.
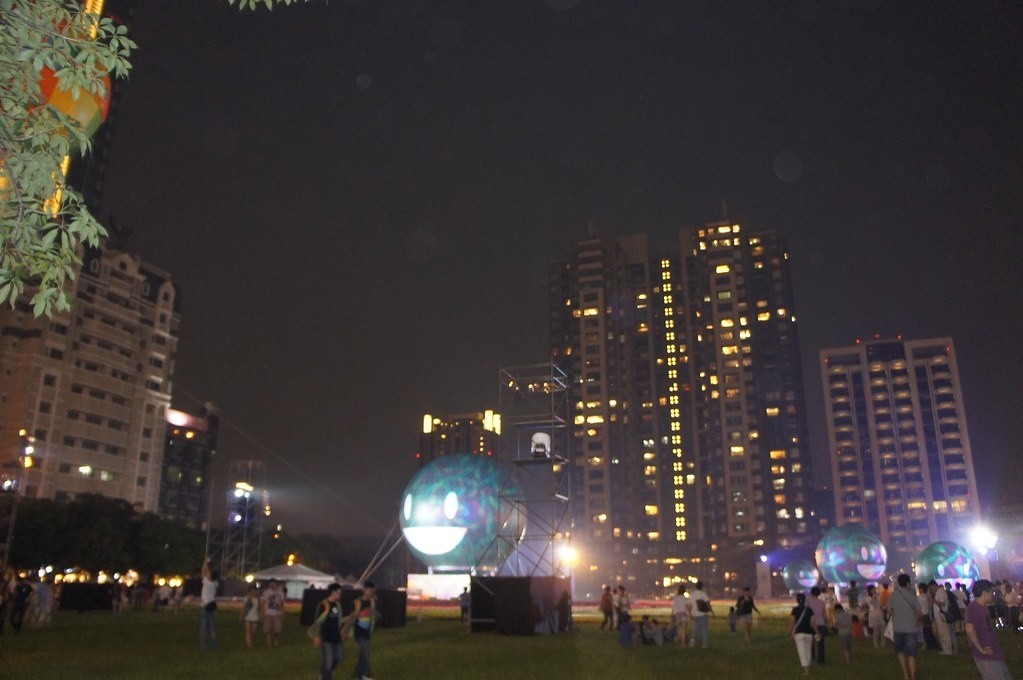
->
[884,616,894,642]
[940,610,955,623]
[306,621,321,641]
[697,599,711,613]
[206,602,218,611]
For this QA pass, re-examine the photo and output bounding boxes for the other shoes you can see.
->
[940,650,950,655]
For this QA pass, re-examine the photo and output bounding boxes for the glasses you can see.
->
[987,590,996,596]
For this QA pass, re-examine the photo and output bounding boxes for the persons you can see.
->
[728,607,735,634]
[987,580,1023,632]
[199,560,218,649]
[0,576,71,635]
[822,580,891,663]
[343,582,382,680]
[109,578,183,615]
[600,581,715,648]
[918,580,970,656]
[457,587,469,618]
[315,583,347,680]
[964,579,1015,680]
[808,587,828,664]
[242,578,288,648]
[791,593,820,676]
[887,574,924,680]
[735,587,760,643]
